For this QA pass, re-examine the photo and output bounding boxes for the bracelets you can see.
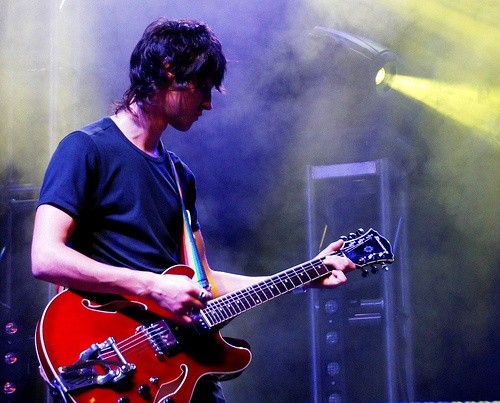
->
[292,285,307,294]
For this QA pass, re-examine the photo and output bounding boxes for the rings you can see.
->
[199,289,206,299]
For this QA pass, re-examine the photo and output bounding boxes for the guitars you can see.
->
[33,228,395,403]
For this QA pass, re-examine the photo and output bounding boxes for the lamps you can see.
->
[311,25,401,97]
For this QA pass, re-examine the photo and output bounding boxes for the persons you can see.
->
[31,16,356,403]
